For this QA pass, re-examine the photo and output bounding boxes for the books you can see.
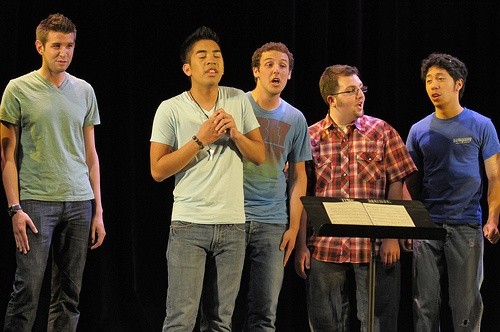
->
[321,201,414,228]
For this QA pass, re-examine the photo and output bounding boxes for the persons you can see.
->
[400,53,500,332]
[149,26,266,332]
[284,63,419,332]
[0,14,106,332]
[244,42,313,332]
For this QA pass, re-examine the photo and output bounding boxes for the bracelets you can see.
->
[192,136,205,149]
[8,205,22,216]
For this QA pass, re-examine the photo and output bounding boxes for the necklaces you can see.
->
[188,88,219,120]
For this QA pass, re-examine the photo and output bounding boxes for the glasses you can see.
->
[330,86,368,95]
